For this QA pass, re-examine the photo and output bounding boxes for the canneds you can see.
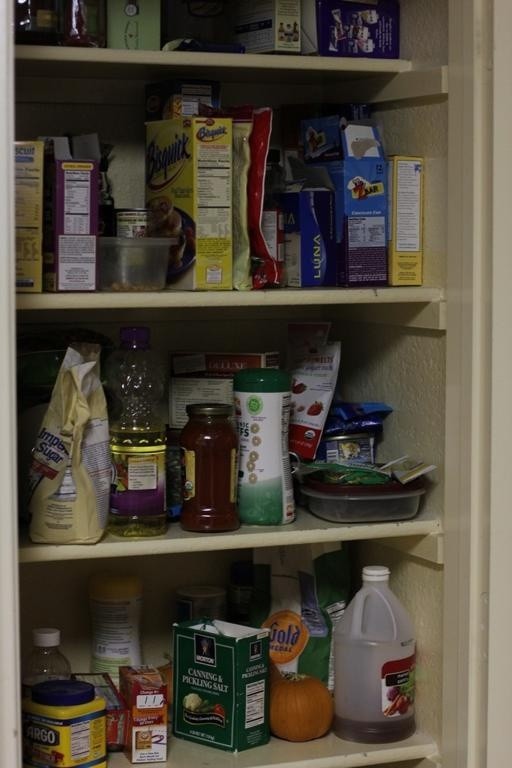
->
[178,403,241,532]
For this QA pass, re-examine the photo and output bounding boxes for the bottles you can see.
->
[24,628,71,692]
[113,324,165,431]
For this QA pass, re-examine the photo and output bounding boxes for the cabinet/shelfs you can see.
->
[0,0,510,763]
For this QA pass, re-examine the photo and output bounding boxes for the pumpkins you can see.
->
[269,672,335,742]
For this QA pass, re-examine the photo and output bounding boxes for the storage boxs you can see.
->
[172,612,274,750]
